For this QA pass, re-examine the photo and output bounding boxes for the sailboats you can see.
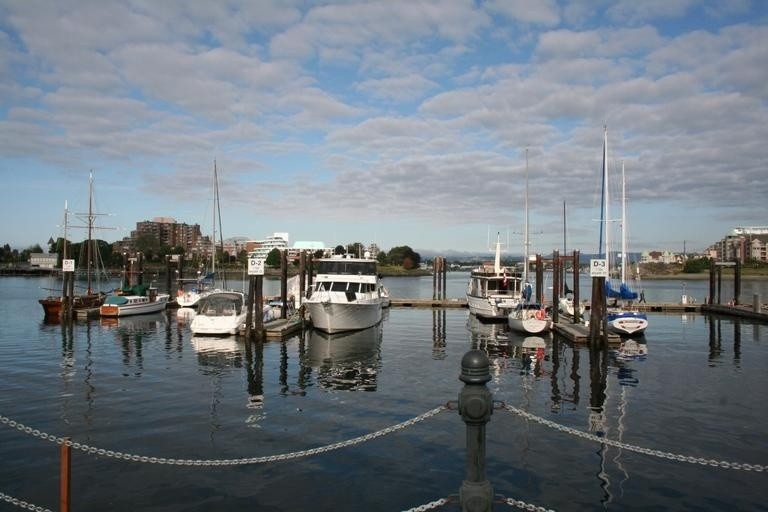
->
[466,125,648,335]
